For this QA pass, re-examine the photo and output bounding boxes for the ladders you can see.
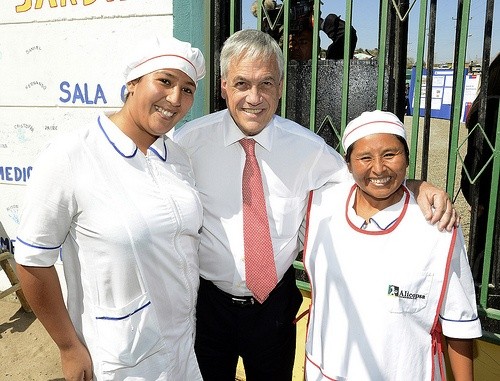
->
[0,247,34,314]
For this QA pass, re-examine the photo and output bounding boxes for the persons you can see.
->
[263,8,357,60]
[172,29,460,381]
[13,35,207,381]
[296,108,484,381]
[459,53,500,310]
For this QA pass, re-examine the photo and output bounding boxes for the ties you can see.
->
[238,136,280,304]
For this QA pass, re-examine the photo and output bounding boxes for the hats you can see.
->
[120,36,207,88]
[341,108,407,150]
